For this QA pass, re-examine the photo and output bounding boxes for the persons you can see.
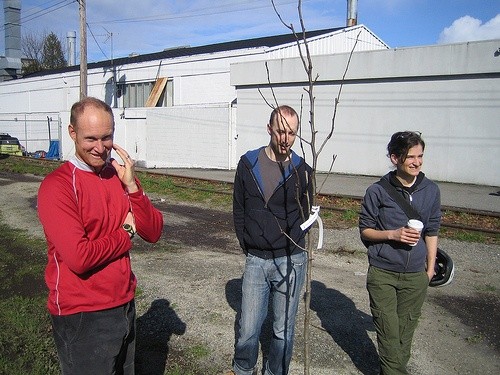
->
[37,97,165,375]
[358,131,442,375]
[233,105,317,375]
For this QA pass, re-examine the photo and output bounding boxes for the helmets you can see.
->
[427,246,455,287]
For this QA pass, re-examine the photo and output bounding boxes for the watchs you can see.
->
[121,223,135,238]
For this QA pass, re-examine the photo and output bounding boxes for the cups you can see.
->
[406,219,424,247]
